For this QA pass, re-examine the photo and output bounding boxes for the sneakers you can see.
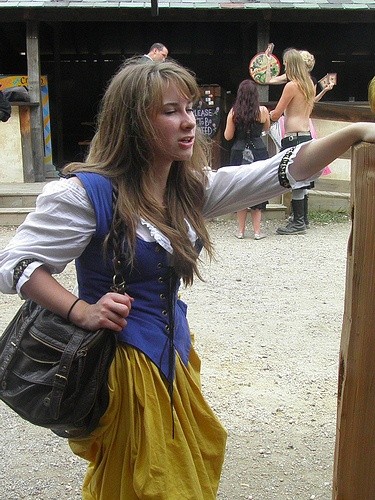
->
[233,229,244,239]
[253,231,268,241]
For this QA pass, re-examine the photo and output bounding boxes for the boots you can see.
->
[275,196,308,235]
[302,194,311,230]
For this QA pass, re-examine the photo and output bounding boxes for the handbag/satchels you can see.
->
[0,297,119,439]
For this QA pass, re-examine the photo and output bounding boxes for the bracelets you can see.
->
[67,298,81,323]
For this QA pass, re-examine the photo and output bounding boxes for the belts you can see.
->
[282,131,311,139]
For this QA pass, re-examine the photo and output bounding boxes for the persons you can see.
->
[0,60,375,500]
[224,79,271,239]
[140,43,170,64]
[265,41,331,235]
